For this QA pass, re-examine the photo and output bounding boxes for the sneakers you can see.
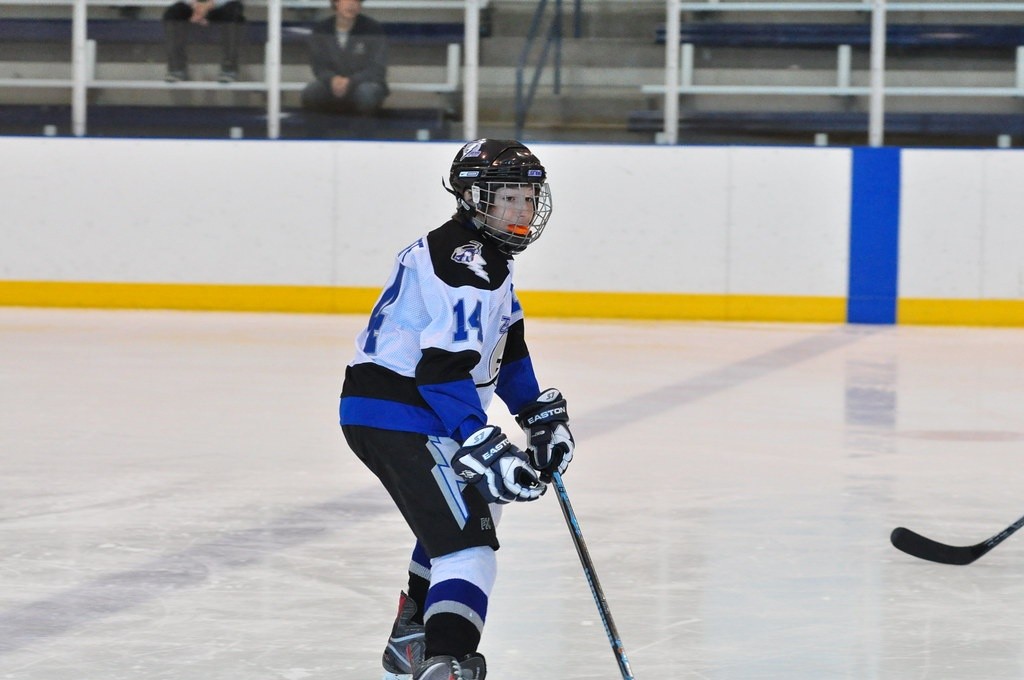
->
[382,590,426,680]
[406,653,487,680]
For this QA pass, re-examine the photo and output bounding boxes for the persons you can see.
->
[341,139,574,680]
[164,0,391,143]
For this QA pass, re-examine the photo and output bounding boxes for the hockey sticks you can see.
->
[885,505,1024,571]
[547,464,640,680]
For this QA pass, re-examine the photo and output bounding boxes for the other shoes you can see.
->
[165,69,187,81]
[218,72,236,83]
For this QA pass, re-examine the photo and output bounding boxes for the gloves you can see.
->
[515,388,575,483]
[451,426,547,504]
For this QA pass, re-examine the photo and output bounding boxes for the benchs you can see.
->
[623,0,1024,149]
[0,0,487,142]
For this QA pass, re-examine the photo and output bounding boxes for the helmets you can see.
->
[442,138,547,217]
